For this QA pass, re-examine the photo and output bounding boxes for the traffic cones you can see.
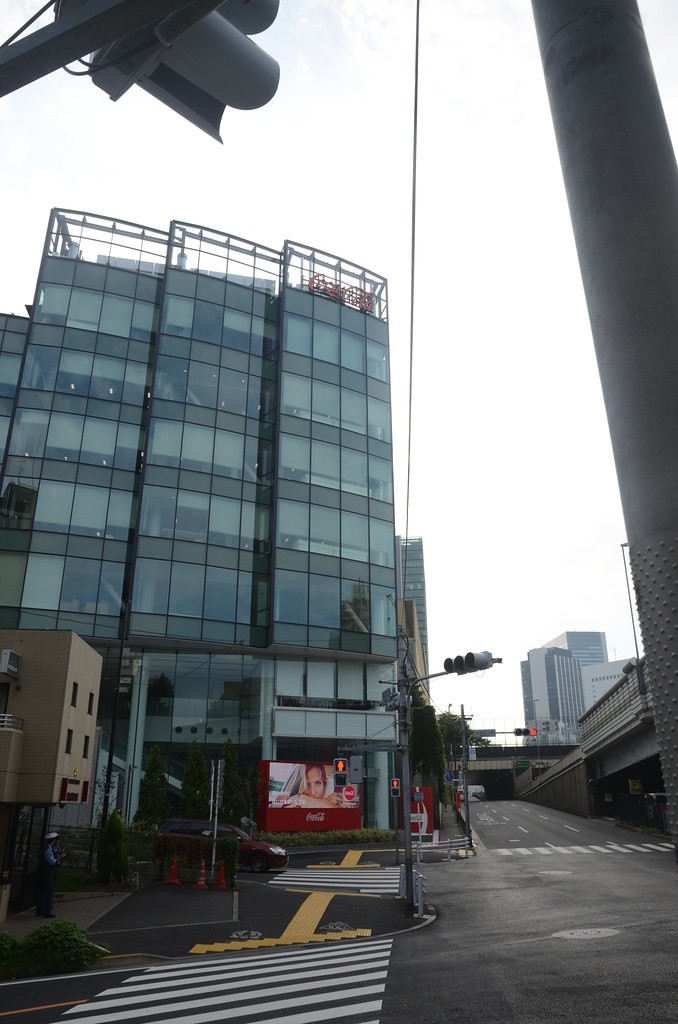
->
[211,860,229,891]
[161,852,180,885]
[190,858,208,890]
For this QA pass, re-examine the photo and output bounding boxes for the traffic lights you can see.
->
[333,757,348,787]
[91,1,281,149]
[514,727,537,737]
[406,683,425,706]
[391,778,400,798]
[445,651,491,676]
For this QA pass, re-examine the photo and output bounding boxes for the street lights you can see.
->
[532,697,542,776]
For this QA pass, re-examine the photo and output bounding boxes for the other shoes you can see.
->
[36,912,43,916]
[44,914,55,918]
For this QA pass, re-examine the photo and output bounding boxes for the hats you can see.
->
[45,832,59,841]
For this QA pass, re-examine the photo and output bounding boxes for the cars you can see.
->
[154,818,290,874]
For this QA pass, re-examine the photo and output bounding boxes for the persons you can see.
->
[269,764,343,808]
[34,832,65,919]
